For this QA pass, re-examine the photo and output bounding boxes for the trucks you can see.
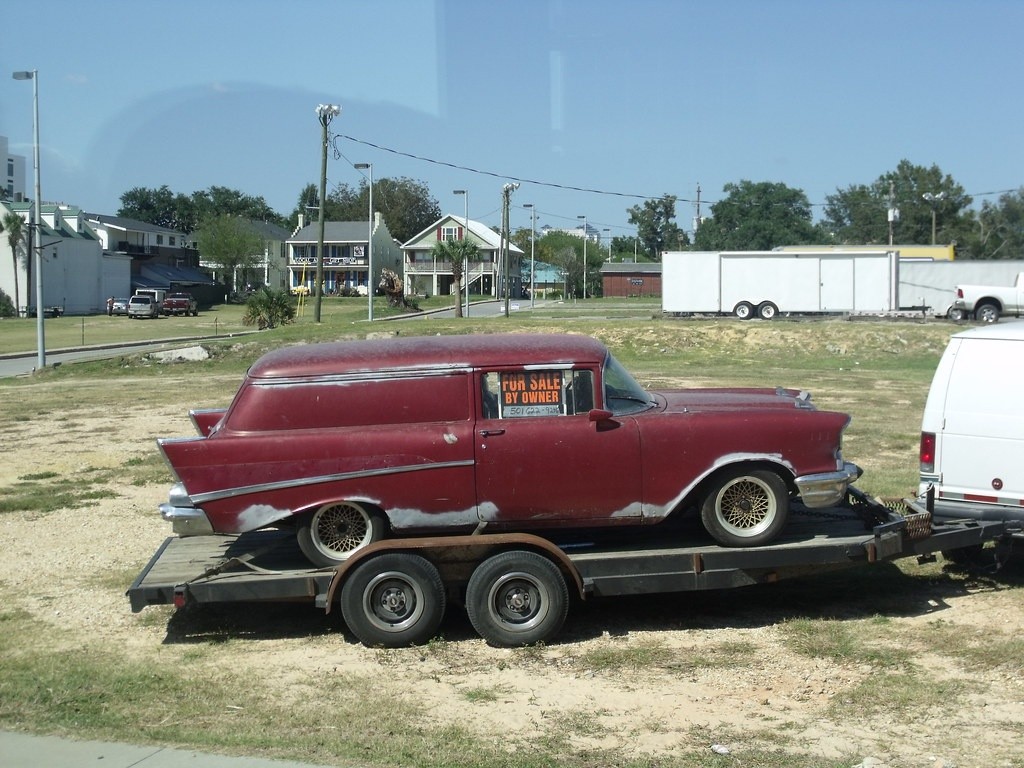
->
[135,290,166,314]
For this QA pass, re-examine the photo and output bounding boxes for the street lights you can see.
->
[603,228,612,263]
[453,189,471,318]
[312,103,343,323]
[12,68,47,369]
[502,181,522,318]
[921,190,947,245]
[523,203,535,309]
[353,162,374,321]
[576,215,587,299]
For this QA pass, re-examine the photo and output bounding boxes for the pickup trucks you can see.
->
[163,293,200,316]
[954,270,1024,324]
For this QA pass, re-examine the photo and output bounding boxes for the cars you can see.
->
[107,298,128,316]
[157,332,866,569]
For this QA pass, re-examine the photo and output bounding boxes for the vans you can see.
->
[917,320,1024,562]
[128,295,160,318]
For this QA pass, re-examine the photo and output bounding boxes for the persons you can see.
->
[106,296,115,316]
